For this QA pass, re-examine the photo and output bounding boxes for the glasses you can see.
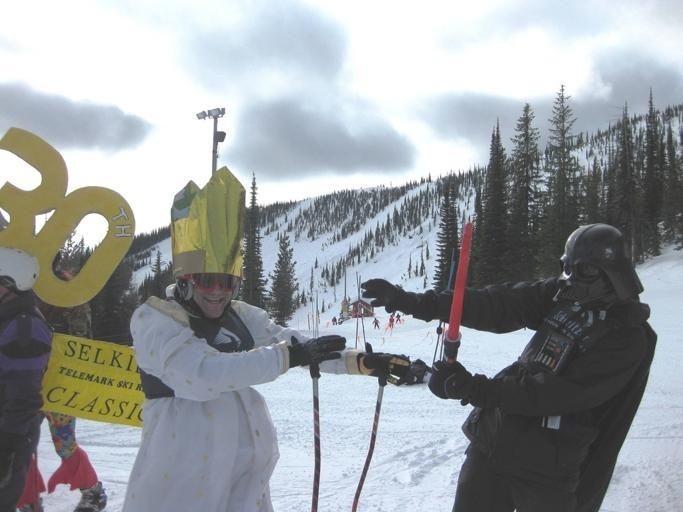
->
[183,273,238,293]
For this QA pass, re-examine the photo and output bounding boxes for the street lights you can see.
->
[196,107,229,171]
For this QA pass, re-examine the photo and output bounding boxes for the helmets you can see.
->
[554,224,642,305]
[0,246,37,291]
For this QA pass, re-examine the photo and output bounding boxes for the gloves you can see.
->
[361,279,422,320]
[430,361,479,406]
[286,335,345,368]
[362,343,408,380]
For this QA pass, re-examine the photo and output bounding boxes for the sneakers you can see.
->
[18,498,45,512]
[73,483,107,512]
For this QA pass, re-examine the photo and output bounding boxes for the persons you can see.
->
[359,221,659,511]
[0,247,54,512]
[394,312,401,324]
[14,250,107,510]
[121,272,411,512]
[388,314,394,329]
[371,317,380,329]
